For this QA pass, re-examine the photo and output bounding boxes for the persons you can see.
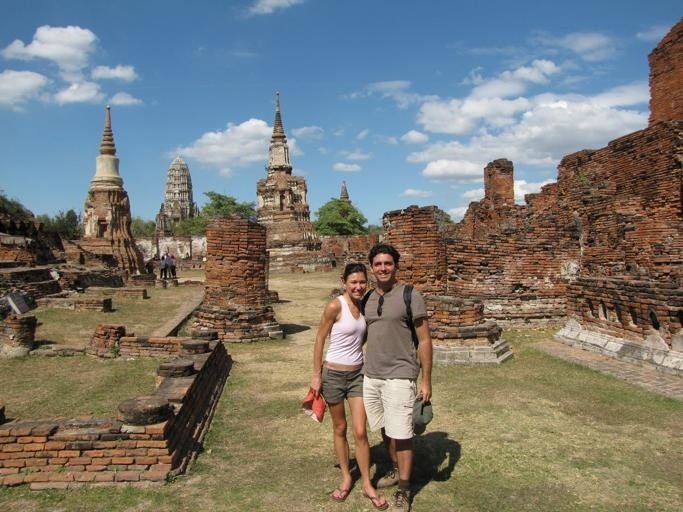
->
[358,243,433,512]
[311,262,390,511]
[144,252,178,278]
[78,251,85,266]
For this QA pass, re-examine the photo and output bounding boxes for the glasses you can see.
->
[377,295,384,317]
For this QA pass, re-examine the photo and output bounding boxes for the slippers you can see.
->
[364,491,389,510]
[332,488,349,501]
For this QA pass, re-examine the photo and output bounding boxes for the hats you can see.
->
[412,395,433,434]
[303,390,326,423]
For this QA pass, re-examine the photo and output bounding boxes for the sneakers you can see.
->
[391,489,411,512]
[374,466,401,488]
